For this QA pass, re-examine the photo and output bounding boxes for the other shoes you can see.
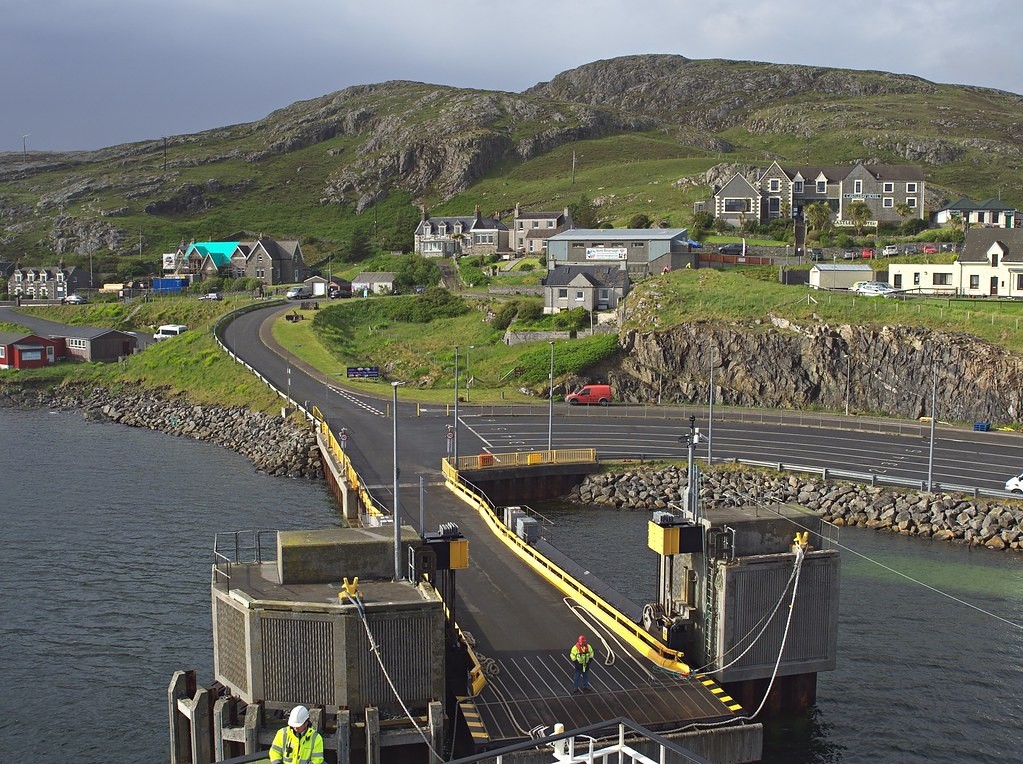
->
[573,686,578,693]
[584,688,592,692]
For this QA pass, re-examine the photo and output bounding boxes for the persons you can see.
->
[686,262,691,269]
[663,266,670,274]
[570,635,594,693]
[269,705,324,764]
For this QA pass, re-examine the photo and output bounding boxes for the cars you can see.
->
[416,286,426,293]
[198,292,223,301]
[862,248,874,258]
[811,248,824,261]
[1005,472,1023,493]
[902,243,961,254]
[882,246,898,256]
[844,250,856,258]
[64,295,87,305]
[330,290,351,298]
[848,281,902,294]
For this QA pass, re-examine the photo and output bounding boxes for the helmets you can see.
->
[288,706,309,727]
[579,636,586,643]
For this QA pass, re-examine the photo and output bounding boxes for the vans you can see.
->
[286,286,313,299]
[153,324,188,342]
[565,384,612,406]
[718,243,748,256]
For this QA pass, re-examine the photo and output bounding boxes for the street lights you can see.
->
[928,359,943,492]
[709,345,717,465]
[391,380,405,579]
[466,345,476,402]
[844,355,850,416]
[548,341,555,463]
